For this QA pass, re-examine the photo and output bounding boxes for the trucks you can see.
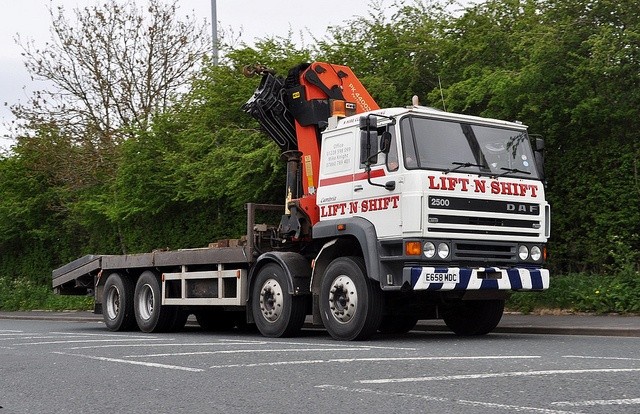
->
[52,61,550,341]
